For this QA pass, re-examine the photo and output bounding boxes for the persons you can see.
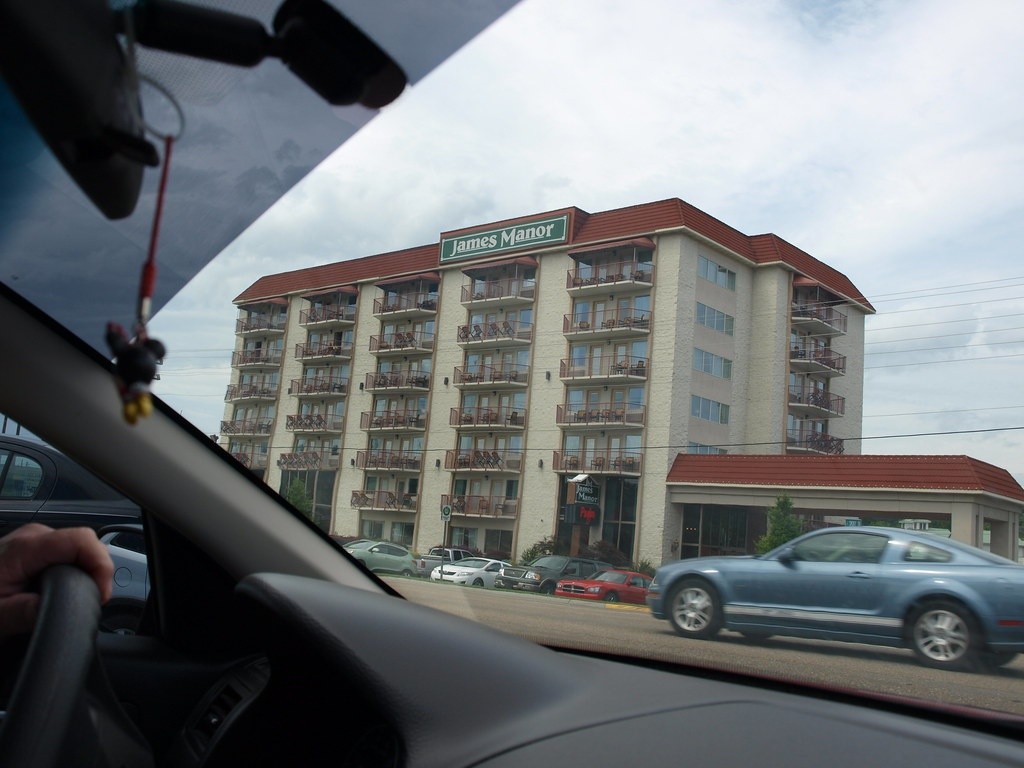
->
[0,523,116,637]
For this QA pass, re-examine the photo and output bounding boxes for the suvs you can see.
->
[494,553,614,597]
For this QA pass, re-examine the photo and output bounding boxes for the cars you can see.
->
[0,433,144,525]
[341,538,417,579]
[430,556,513,589]
[645,525,1024,673]
[554,567,654,606]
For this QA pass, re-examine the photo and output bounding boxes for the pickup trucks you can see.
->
[413,548,476,579]
